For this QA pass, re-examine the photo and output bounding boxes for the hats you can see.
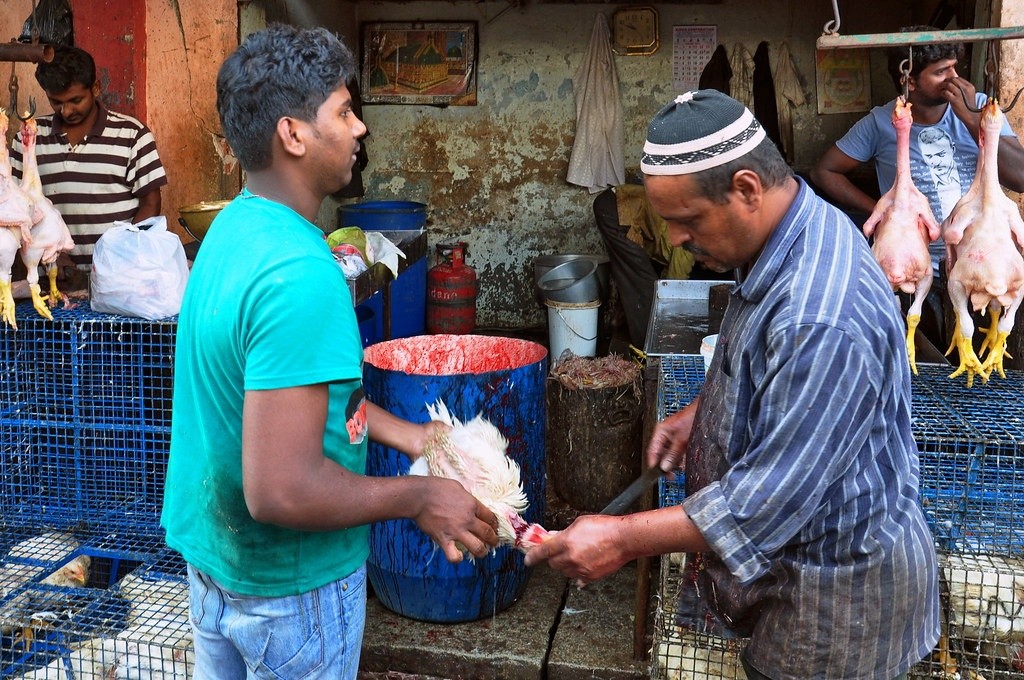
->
[640,88,766,176]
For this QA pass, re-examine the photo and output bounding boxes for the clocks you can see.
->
[609,5,660,56]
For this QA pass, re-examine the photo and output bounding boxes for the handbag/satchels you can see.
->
[90,215,192,320]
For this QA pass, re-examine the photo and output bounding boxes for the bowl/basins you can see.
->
[533,254,611,295]
[537,258,603,303]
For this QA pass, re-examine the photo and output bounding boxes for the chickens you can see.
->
[862,97,944,373]
[13,110,91,323]
[0,531,95,652]
[946,530,1024,671]
[19,573,194,680]
[0,107,43,332]
[407,398,558,565]
[940,97,1024,388]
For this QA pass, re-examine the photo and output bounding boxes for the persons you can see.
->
[809,23,1023,368]
[525,88,942,679]
[12,46,168,281]
[161,25,500,679]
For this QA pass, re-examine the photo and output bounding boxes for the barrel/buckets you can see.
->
[354,305,378,350]
[545,297,602,363]
[336,200,428,342]
[361,334,550,625]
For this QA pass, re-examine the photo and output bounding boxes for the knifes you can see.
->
[598,458,667,516]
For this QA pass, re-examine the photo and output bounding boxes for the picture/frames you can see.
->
[359,19,478,106]
